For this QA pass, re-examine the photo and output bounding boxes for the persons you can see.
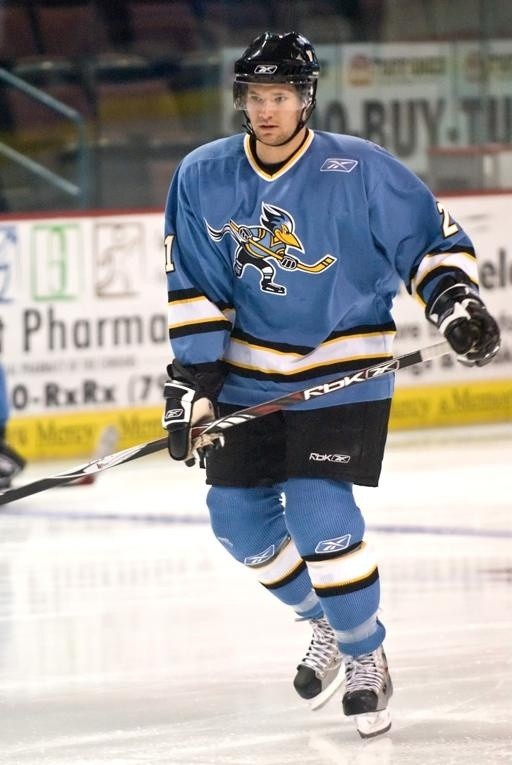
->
[159,29,505,718]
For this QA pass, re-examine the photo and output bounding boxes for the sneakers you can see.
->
[292,615,343,700]
[340,645,394,716]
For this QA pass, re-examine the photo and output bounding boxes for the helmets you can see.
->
[233,30,321,111]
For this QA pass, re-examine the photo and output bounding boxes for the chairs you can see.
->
[1,0,511,208]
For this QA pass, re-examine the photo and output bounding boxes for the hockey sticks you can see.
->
[8,427,118,487]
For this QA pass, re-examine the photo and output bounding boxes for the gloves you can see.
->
[428,283,504,376]
[160,359,222,467]
